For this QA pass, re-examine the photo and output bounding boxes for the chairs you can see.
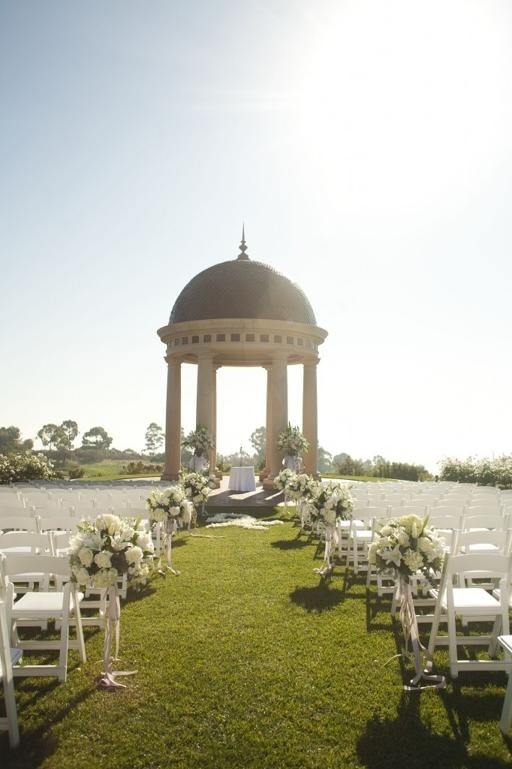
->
[2,557,127,749]
[2,480,183,598]
[314,479,510,595]
[389,551,512,736]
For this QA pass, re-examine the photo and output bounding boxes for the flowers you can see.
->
[274,468,313,502]
[275,420,310,465]
[180,424,216,461]
[180,472,211,525]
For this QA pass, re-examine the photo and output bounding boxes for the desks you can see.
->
[230,466,254,492]
